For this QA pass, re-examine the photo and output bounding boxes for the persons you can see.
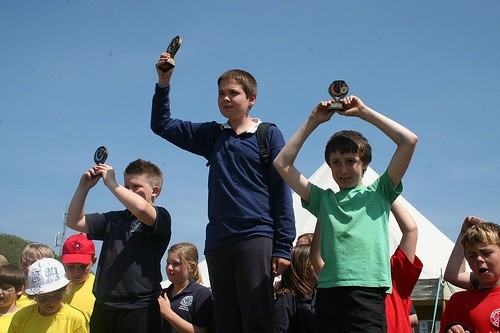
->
[15,243,55,310]
[7,258,88,333]
[297,233,314,245]
[385,199,423,333]
[409,304,418,327]
[443,216,485,289]
[60,233,98,324]
[150,51,296,333]
[439,222,500,333]
[273,94,418,333]
[66,158,172,333]
[273,243,318,333]
[158,242,215,333]
[0,264,27,333]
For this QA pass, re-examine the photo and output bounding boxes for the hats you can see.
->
[25,257,70,295]
[61,232,95,264]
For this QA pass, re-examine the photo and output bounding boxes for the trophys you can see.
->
[157,36,183,73]
[326,80,349,110]
[91,146,109,178]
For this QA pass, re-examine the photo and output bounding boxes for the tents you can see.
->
[159,160,474,333]
